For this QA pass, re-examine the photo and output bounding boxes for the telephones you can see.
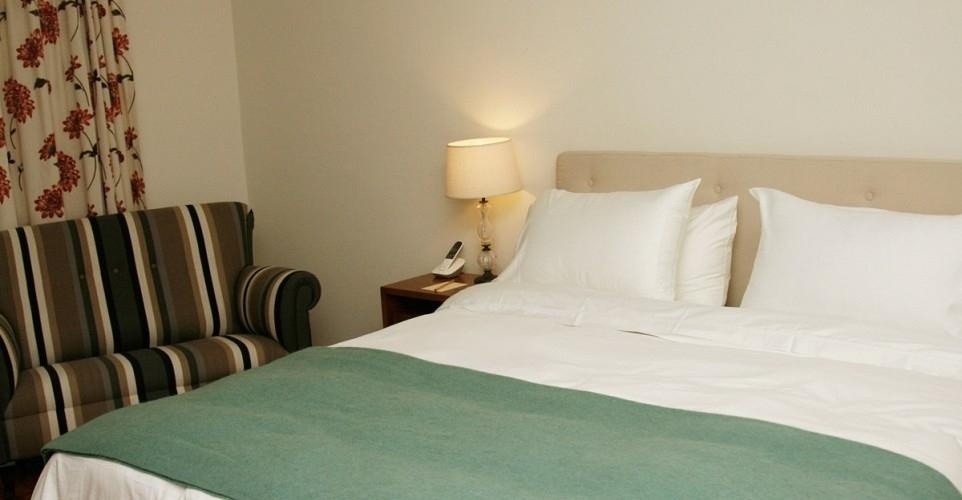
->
[432,241,465,277]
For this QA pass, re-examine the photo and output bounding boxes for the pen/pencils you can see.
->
[434,278,456,291]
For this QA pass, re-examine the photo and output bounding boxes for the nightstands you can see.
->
[380,273,480,326]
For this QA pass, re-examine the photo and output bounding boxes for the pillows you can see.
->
[738,185,960,322]
[493,177,703,302]
[672,195,740,309]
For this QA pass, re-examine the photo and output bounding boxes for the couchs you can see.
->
[0,201,321,468]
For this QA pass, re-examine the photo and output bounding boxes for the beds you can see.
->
[40,151,961,500]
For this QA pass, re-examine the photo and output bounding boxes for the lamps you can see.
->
[446,137,524,284]
